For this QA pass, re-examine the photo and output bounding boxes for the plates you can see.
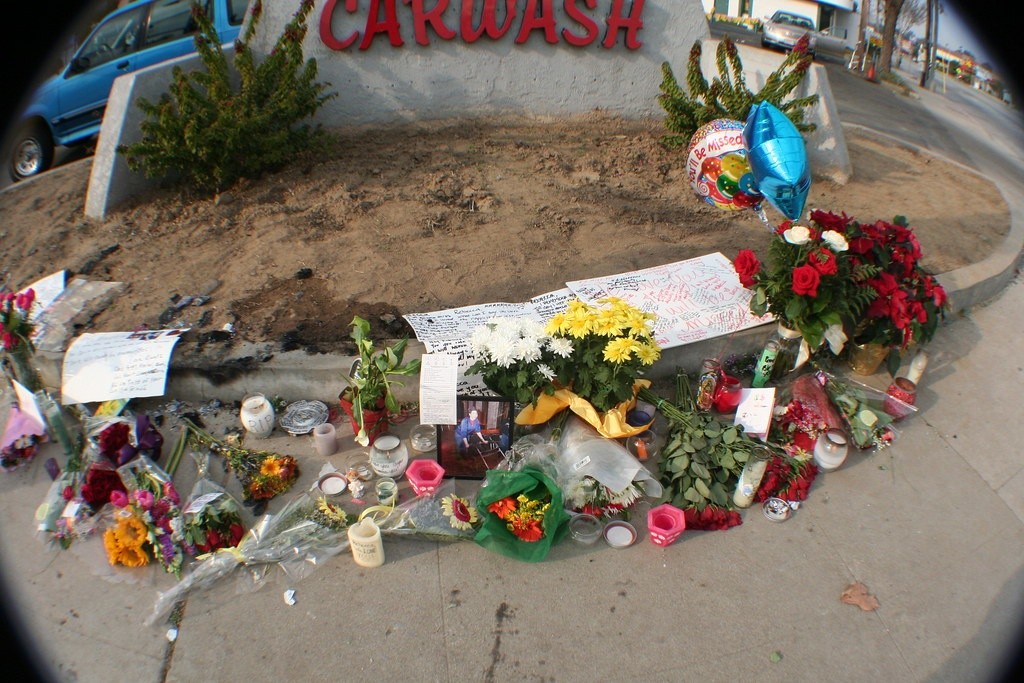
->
[279,399,329,435]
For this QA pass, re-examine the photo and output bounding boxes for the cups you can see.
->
[313,423,337,457]
[647,504,686,547]
[405,459,445,496]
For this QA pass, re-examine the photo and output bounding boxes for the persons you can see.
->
[453,408,509,462]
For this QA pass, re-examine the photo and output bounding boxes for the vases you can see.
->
[696,319,917,424]
[341,451,375,486]
[4,343,45,393]
[369,433,409,482]
[406,458,446,497]
[408,422,438,454]
[813,427,849,475]
[340,386,389,445]
[732,446,771,510]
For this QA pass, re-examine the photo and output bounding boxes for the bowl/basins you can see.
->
[628,430,657,462]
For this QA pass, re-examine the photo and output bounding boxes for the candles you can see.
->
[634,437,649,462]
[906,349,928,383]
[347,522,386,568]
[312,422,340,456]
[373,478,399,508]
[240,393,275,441]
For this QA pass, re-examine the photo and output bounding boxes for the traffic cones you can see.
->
[864,64,875,81]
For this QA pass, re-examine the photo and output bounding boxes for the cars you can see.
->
[761,10,847,58]
[7,0,244,183]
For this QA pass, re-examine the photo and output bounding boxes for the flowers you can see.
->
[850,217,950,348]
[342,314,421,448]
[558,413,664,521]
[488,491,551,543]
[732,198,849,356]
[28,412,478,587]
[0,284,36,353]
[653,399,910,534]
[470,289,664,397]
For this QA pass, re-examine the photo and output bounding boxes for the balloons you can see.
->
[686,101,811,234]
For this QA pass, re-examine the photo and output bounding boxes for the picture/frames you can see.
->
[436,396,515,482]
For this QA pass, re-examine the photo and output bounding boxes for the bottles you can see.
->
[713,376,742,414]
[34,391,80,466]
[907,348,930,383]
[409,424,437,452]
[748,339,781,388]
[369,432,408,480]
[373,477,399,507]
[567,514,604,549]
[695,360,721,411]
[344,451,375,480]
[884,375,917,423]
[732,445,771,509]
[348,522,385,568]
[240,391,274,439]
[812,427,848,473]
